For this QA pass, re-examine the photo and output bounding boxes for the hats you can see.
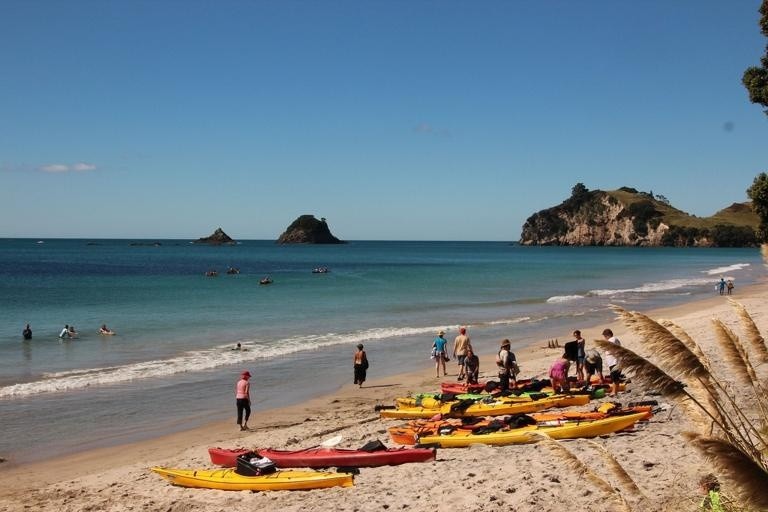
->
[437,331,444,336]
[587,354,597,364]
[241,372,251,377]
[500,339,510,346]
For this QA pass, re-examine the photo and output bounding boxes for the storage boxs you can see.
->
[234,450,277,476]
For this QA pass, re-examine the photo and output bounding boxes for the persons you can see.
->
[726,280,732,294]
[233,371,251,430]
[548,329,621,397]
[59,324,68,338]
[235,343,241,350]
[495,340,512,391]
[102,324,108,332]
[509,351,516,388]
[716,277,727,294]
[23,325,32,340]
[453,328,471,381]
[354,344,369,387]
[68,326,78,339]
[464,350,479,384]
[432,330,448,378]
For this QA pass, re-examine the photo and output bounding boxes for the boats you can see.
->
[151,466,361,491]
[99,328,116,336]
[378,379,662,449]
[206,270,218,277]
[260,279,274,285]
[312,269,332,274]
[227,269,241,275]
[208,440,437,468]
